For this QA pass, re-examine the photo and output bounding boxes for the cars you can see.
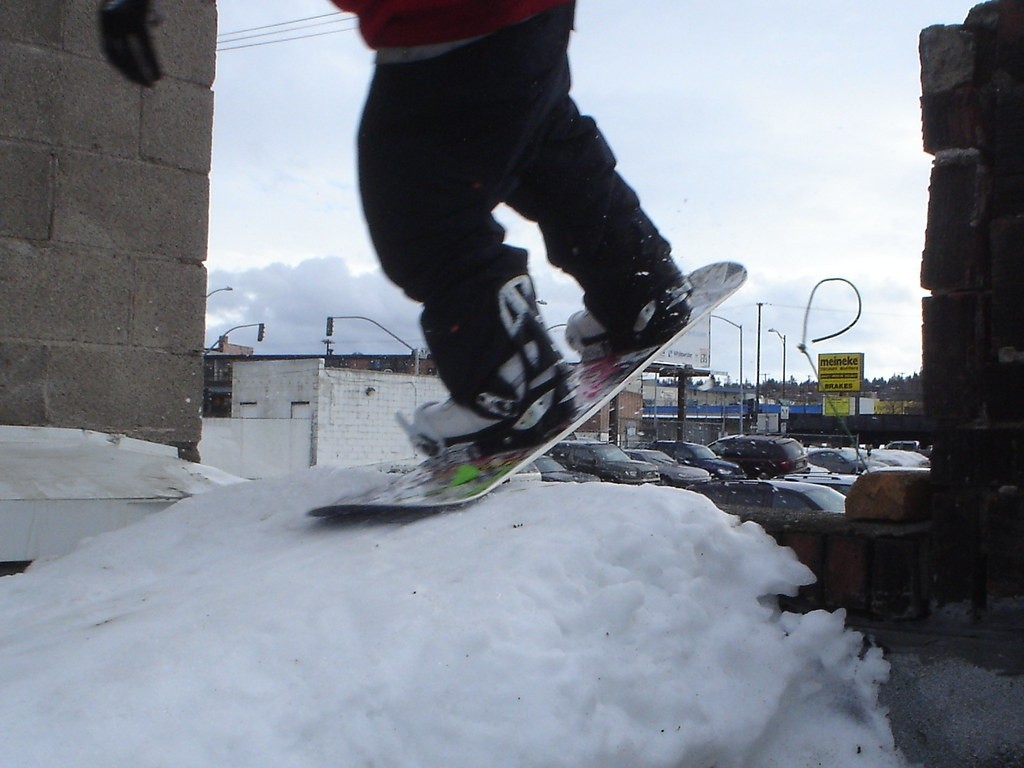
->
[498,432,933,486]
[686,477,847,516]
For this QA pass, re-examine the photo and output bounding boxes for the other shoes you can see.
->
[414,370,578,456]
[564,284,690,356]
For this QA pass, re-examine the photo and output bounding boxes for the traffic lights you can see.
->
[326,318,333,334]
[258,323,266,342]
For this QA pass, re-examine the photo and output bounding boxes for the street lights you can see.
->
[768,328,786,400]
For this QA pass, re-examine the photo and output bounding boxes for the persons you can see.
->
[96,0,692,453]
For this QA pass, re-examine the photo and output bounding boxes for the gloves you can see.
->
[98,1,163,88]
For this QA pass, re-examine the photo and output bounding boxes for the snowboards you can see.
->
[302,261,749,525]
